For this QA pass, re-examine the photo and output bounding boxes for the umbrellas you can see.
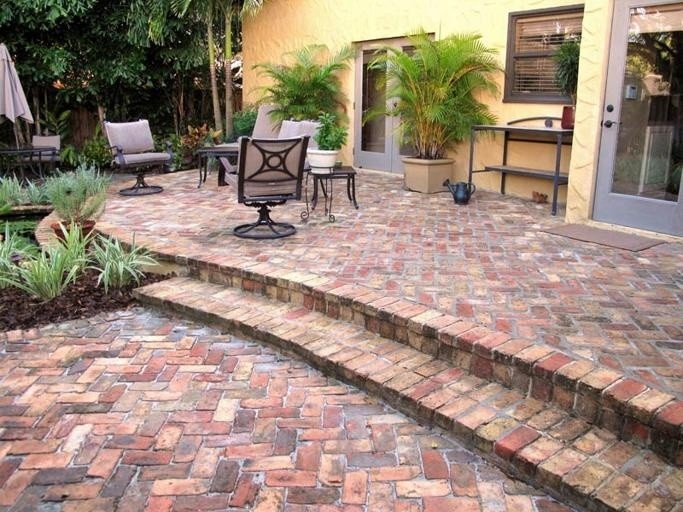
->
[0,42,35,183]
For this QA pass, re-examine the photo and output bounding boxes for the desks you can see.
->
[309,166,360,210]
[1,147,57,187]
[196,142,239,189]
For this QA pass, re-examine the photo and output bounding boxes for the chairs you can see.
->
[25,135,61,177]
[211,104,294,187]
[278,120,321,172]
[220,134,312,240]
[104,118,170,197]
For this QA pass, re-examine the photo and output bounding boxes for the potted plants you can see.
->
[360,25,508,193]
[41,163,114,255]
[307,111,350,174]
[551,38,580,129]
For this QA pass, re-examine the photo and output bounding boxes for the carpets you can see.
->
[540,224,666,252]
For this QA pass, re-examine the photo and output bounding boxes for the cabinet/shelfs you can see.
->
[468,125,573,217]
